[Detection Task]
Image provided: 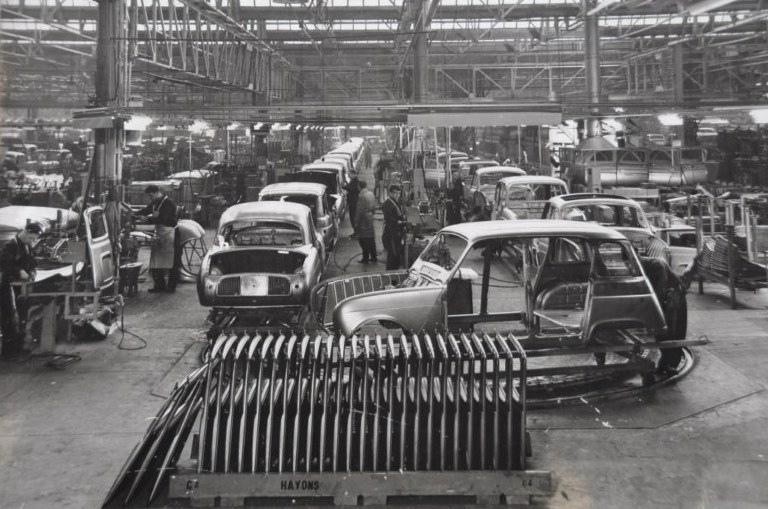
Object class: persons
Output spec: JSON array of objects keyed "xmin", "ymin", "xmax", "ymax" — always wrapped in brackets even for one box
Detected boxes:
[
  {"xmin": 344, "ymin": 143, "xmax": 405, "ymax": 271},
  {"xmin": 0, "ymin": 221, "xmax": 42, "ymax": 346},
  {"xmin": 133, "ymin": 184, "xmax": 177, "ymax": 294},
  {"xmin": 622, "ymin": 245, "xmax": 688, "ymax": 379}
]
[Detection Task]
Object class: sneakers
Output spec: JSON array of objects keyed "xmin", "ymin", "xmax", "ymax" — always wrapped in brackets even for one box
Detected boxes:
[
  {"xmin": 357, "ymin": 257, "xmax": 378, "ymax": 265},
  {"xmin": 148, "ymin": 284, "xmax": 176, "ymax": 294}
]
